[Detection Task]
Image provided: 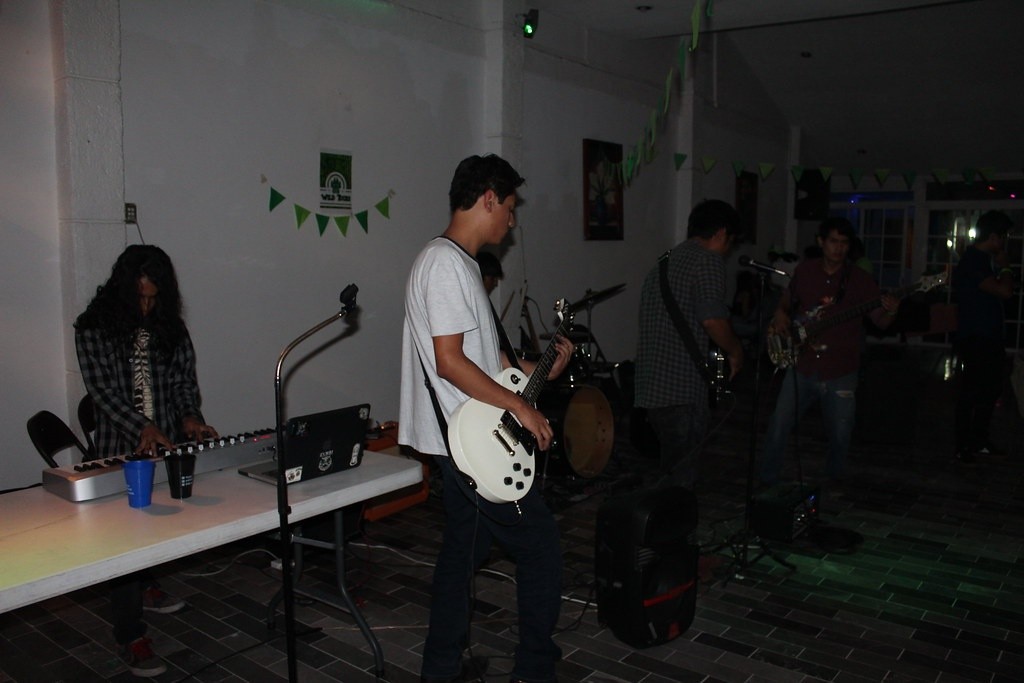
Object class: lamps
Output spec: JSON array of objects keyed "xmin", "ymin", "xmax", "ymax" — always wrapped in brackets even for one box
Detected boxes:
[{"xmin": 520, "ymin": 7, "xmax": 538, "ymax": 39}]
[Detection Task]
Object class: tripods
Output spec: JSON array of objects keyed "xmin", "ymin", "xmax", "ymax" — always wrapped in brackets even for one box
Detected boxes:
[{"xmin": 699, "ymin": 269, "xmax": 797, "ymax": 588}]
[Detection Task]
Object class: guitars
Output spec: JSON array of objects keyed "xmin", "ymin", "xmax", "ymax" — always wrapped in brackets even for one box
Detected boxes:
[
  {"xmin": 445, "ymin": 295, "xmax": 576, "ymax": 503},
  {"xmin": 706, "ymin": 343, "xmax": 735, "ymax": 414},
  {"xmin": 765, "ymin": 269, "xmax": 953, "ymax": 370}
]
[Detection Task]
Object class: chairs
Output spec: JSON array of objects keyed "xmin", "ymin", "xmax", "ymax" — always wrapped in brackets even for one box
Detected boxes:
[
  {"xmin": 78, "ymin": 394, "xmax": 96, "ymax": 454},
  {"xmin": 27, "ymin": 411, "xmax": 89, "ymax": 469}
]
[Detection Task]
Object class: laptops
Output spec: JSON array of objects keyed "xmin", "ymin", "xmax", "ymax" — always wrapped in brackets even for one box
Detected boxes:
[{"xmin": 238, "ymin": 403, "xmax": 372, "ymax": 486}]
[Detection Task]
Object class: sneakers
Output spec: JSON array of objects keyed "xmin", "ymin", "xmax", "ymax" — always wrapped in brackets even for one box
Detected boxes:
[
  {"xmin": 115, "ymin": 636, "xmax": 167, "ymax": 677},
  {"xmin": 421, "ymin": 655, "xmax": 490, "ymax": 683},
  {"xmin": 955, "ymin": 447, "xmax": 975, "ymax": 462},
  {"xmin": 142, "ymin": 586, "xmax": 185, "ymax": 613},
  {"xmin": 510, "ymin": 679, "xmax": 558, "ymax": 683},
  {"xmin": 971, "ymin": 442, "xmax": 1008, "ymax": 459}
]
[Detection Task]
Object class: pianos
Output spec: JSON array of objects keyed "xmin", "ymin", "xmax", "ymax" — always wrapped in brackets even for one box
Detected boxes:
[{"xmin": 42, "ymin": 422, "xmax": 296, "ymax": 500}]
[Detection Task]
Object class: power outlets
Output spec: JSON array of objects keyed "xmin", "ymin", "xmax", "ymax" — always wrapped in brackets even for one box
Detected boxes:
[{"xmin": 125, "ymin": 203, "xmax": 137, "ymax": 223}]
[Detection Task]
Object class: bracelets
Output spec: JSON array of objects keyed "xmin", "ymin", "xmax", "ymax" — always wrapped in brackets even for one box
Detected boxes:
[
  {"xmin": 1000, "ymin": 268, "xmax": 1013, "ymax": 274},
  {"xmin": 887, "ymin": 310, "xmax": 898, "ymax": 316}
]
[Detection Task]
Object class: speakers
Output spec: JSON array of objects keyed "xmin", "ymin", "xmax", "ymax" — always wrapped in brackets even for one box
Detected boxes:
[
  {"xmin": 596, "ymin": 484, "xmax": 699, "ymax": 648},
  {"xmin": 793, "ymin": 169, "xmax": 831, "ymax": 221}
]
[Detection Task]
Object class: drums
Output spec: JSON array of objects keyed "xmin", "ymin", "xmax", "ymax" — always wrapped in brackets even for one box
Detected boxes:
[
  {"xmin": 585, "ymin": 362, "xmax": 622, "ymax": 401},
  {"xmin": 536, "ymin": 383, "xmax": 616, "ymax": 479}
]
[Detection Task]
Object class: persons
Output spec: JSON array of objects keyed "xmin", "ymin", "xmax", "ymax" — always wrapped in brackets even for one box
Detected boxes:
[
  {"xmin": 950, "ymin": 209, "xmax": 1014, "ymax": 462},
  {"xmin": 74, "ymin": 245, "xmax": 220, "ymax": 677},
  {"xmin": 634, "ymin": 200, "xmax": 901, "ymax": 546},
  {"xmin": 397, "ymin": 154, "xmax": 576, "ymax": 683}
]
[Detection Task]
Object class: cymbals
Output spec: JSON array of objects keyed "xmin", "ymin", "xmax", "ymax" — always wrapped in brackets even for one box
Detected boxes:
[{"xmin": 566, "ymin": 284, "xmax": 628, "ymax": 314}]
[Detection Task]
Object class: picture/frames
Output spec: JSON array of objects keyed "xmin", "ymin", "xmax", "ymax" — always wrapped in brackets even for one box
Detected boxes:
[
  {"xmin": 736, "ymin": 170, "xmax": 759, "ymax": 244},
  {"xmin": 583, "ymin": 138, "xmax": 624, "ymax": 241}
]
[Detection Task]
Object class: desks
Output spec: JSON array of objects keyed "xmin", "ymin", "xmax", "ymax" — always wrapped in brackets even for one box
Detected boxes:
[
  {"xmin": 0, "ymin": 450, "xmax": 423, "ymax": 678},
  {"xmin": 365, "ymin": 420, "xmax": 430, "ymax": 521}
]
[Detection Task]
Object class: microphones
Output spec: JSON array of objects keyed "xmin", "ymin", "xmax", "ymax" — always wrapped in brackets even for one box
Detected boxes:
[{"xmin": 738, "ymin": 254, "xmax": 789, "ymax": 276}]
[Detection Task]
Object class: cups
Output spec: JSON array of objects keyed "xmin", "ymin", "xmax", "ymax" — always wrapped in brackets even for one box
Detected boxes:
[
  {"xmin": 163, "ymin": 454, "xmax": 196, "ymax": 499},
  {"xmin": 122, "ymin": 460, "xmax": 156, "ymax": 508}
]
